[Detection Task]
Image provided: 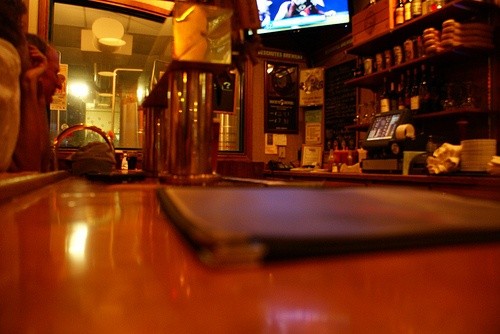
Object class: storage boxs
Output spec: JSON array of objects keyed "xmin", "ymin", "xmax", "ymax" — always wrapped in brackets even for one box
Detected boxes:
[{"xmin": 460, "ymin": 137, "xmax": 497, "ymax": 173}]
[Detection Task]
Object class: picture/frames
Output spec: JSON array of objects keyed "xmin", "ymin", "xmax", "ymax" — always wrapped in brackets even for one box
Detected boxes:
[
  {"xmin": 362, "ymin": 108, "xmax": 411, "ymax": 148},
  {"xmin": 263, "ymin": 59, "xmax": 300, "ymax": 135}
]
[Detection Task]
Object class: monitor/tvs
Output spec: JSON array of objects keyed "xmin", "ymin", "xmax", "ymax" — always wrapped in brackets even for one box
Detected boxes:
[
  {"xmin": 255, "ymin": 0, "xmax": 351, "ymax": 34},
  {"xmin": 364, "ymin": 109, "xmax": 405, "ymax": 143}
]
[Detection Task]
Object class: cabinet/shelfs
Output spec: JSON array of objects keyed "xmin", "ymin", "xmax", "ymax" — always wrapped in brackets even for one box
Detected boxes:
[{"xmin": 345, "ymin": 0, "xmax": 492, "ymax": 133}]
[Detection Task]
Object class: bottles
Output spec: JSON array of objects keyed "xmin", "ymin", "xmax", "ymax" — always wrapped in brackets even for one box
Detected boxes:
[
  {"xmin": 121, "ymin": 151, "xmax": 128, "ymax": 169},
  {"xmin": 328, "ymin": 148, "xmax": 338, "ymax": 172},
  {"xmin": 379, "ymin": 61, "xmax": 441, "ymax": 114},
  {"xmin": 396, "ymin": 0, "xmax": 445, "ymax": 26},
  {"xmin": 363, "ymin": 32, "xmax": 425, "ymax": 75},
  {"xmin": 441, "ymin": 82, "xmax": 480, "ymax": 110}
]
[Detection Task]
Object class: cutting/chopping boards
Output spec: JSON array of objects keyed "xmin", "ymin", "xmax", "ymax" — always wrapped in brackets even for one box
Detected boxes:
[{"xmin": 424, "ymin": 19, "xmax": 493, "ymax": 52}]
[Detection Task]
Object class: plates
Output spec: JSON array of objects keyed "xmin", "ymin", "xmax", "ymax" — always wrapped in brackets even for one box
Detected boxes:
[{"xmin": 459, "ymin": 139, "xmax": 497, "ymax": 172}]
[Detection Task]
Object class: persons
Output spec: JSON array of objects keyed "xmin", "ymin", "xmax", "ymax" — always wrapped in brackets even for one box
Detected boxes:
[
  {"xmin": 0, "ymin": 0, "xmax": 64, "ymax": 176},
  {"xmin": 256, "ymin": 0, "xmax": 336, "ymax": 28}
]
